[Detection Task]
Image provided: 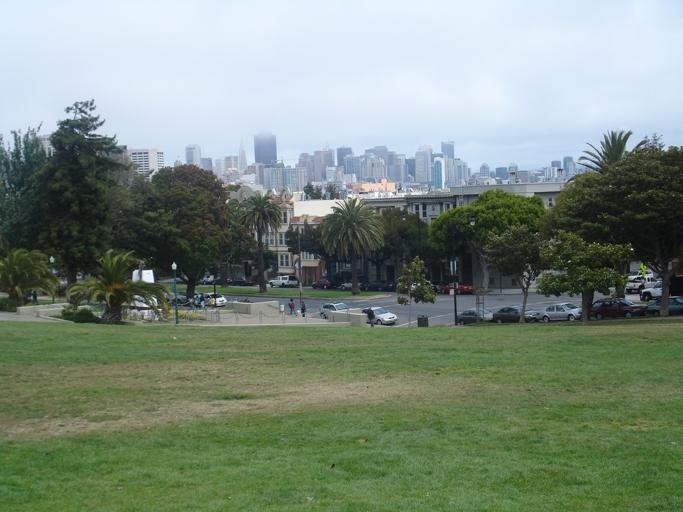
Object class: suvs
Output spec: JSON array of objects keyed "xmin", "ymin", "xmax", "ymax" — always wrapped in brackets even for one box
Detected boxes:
[{"xmin": 269, "ymin": 275, "xmax": 298, "ymax": 288}]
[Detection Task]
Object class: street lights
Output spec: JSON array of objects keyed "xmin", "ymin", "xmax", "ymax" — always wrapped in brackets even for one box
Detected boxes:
[
  {"xmin": 171, "ymin": 261, "xmax": 179, "ymax": 324},
  {"xmin": 49, "ymin": 256, "xmax": 55, "ymax": 303}
]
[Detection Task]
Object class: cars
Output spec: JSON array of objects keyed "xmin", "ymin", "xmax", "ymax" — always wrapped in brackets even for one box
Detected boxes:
[
  {"xmin": 176, "ymin": 278, "xmax": 253, "ymax": 286},
  {"xmin": 311, "ymin": 278, "xmax": 473, "ymax": 294},
  {"xmin": 165, "ymin": 291, "xmax": 227, "ymax": 307},
  {"xmin": 588, "ymin": 269, "xmax": 682, "ymax": 320},
  {"xmin": 362, "ymin": 306, "xmax": 397, "ymax": 325},
  {"xmin": 457, "ymin": 303, "xmax": 582, "ymax": 325},
  {"xmin": 320, "ymin": 303, "xmax": 352, "ymax": 320}
]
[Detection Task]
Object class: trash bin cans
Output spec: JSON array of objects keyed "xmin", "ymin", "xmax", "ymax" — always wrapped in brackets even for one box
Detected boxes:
[
  {"xmin": 280, "ymin": 305, "xmax": 284, "ymax": 312},
  {"xmin": 449, "ymin": 288, "xmax": 454, "ymax": 296},
  {"xmin": 418, "ymin": 314, "xmax": 428, "ymax": 327}
]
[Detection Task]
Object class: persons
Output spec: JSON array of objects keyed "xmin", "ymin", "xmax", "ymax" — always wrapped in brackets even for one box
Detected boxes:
[
  {"xmin": 366, "ymin": 307, "xmax": 375, "ymax": 326},
  {"xmin": 287, "ymin": 298, "xmax": 297, "ymax": 315},
  {"xmin": 300, "ymin": 301, "xmax": 306, "ymax": 317}
]
